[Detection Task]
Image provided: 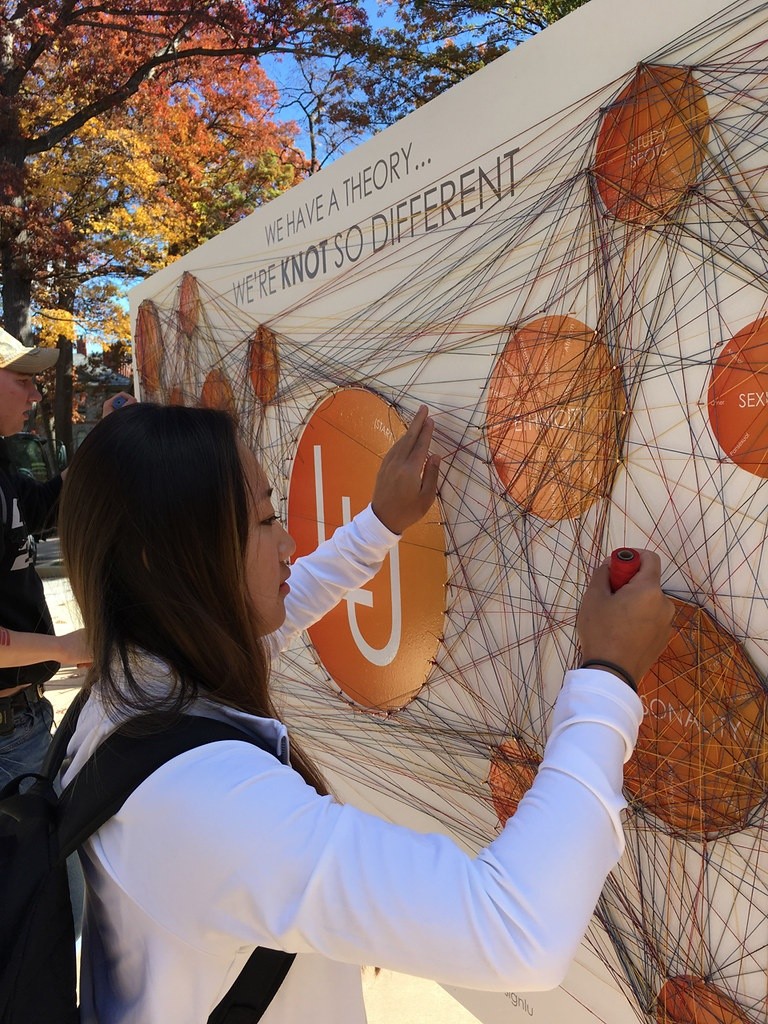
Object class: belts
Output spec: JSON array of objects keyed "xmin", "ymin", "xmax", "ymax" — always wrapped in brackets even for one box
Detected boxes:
[{"xmin": 0, "ymin": 680, "xmax": 47, "ymax": 713}]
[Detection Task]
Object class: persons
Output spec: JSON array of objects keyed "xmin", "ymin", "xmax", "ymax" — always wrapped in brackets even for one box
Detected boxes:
[
  {"xmin": 0, "ymin": 320, "xmax": 95, "ymax": 1024},
  {"xmin": 55, "ymin": 402, "xmax": 677, "ymax": 1024}
]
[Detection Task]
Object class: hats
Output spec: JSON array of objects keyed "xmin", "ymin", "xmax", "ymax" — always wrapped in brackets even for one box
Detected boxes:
[{"xmin": 0, "ymin": 326, "xmax": 60, "ymax": 373}]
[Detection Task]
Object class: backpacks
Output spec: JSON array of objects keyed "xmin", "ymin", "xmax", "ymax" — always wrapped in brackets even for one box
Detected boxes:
[{"xmin": 0, "ymin": 672, "xmax": 318, "ymax": 1024}]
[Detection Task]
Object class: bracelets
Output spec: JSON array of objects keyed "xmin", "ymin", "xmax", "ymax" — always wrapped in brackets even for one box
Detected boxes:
[{"xmin": 581, "ymin": 659, "xmax": 638, "ymax": 693}]
